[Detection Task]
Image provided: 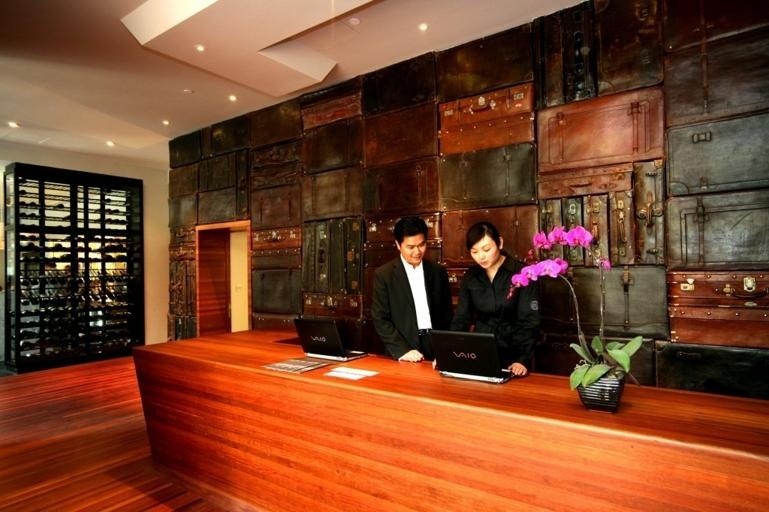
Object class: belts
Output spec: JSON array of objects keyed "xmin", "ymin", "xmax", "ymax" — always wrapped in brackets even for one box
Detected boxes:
[{"xmin": 417, "ymin": 328, "xmax": 434, "ymax": 338}]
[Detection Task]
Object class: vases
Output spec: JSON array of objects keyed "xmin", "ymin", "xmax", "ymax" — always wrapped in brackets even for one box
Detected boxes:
[{"xmin": 577, "ymin": 375, "xmax": 625, "ymax": 414}]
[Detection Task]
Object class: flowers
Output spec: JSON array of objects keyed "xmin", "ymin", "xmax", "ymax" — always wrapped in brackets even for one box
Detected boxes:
[{"xmin": 507, "ymin": 225, "xmax": 643, "ymax": 392}]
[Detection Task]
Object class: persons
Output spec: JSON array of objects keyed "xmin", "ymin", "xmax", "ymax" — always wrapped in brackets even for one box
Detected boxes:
[
  {"xmin": 448, "ymin": 220, "xmax": 543, "ymax": 377},
  {"xmin": 369, "ymin": 215, "xmax": 455, "ymax": 364}
]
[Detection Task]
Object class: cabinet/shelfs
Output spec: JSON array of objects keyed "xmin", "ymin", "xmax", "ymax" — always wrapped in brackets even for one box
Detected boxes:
[{"xmin": 5, "ymin": 162, "xmax": 144, "ymax": 375}]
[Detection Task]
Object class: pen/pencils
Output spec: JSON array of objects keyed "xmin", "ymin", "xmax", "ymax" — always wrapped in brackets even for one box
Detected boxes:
[{"xmin": 501, "ymin": 368, "xmax": 512, "ymax": 372}]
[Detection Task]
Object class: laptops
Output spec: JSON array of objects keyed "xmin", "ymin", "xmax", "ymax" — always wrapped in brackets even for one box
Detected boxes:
[
  {"xmin": 432, "ymin": 330, "xmax": 514, "ymax": 384},
  {"xmin": 293, "ymin": 319, "xmax": 368, "ymax": 361}
]
